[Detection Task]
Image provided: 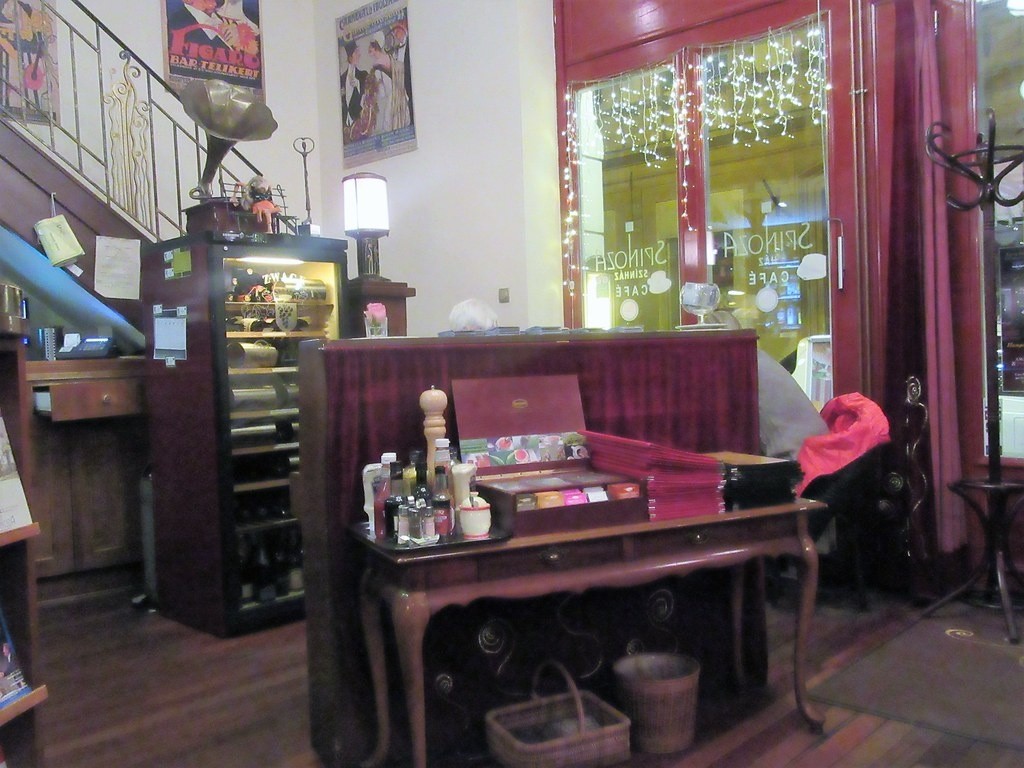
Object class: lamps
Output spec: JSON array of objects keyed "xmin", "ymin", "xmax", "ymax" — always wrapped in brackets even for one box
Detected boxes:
[{"xmin": 342, "ymin": 172, "xmax": 392, "ymax": 282}]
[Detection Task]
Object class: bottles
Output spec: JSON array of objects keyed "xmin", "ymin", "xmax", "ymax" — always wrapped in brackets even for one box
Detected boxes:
[
  {"xmin": 384, "ymin": 461, "xmax": 407, "ymax": 541},
  {"xmin": 431, "ymin": 466, "xmax": 455, "ymax": 536},
  {"xmin": 399, "ymin": 508, "xmax": 409, "ymax": 538},
  {"xmin": 372, "ymin": 453, "xmax": 396, "ymax": 540},
  {"xmin": 424, "ymin": 506, "xmax": 435, "ymax": 536},
  {"xmin": 412, "ymin": 460, "xmax": 432, "ymax": 499},
  {"xmin": 402, "ymin": 451, "xmax": 431, "ymax": 496},
  {"xmin": 431, "ymin": 494, "xmax": 451, "ymax": 538},
  {"xmin": 434, "ymin": 438, "xmax": 451, "ymax": 489},
  {"xmin": 236, "ymin": 530, "xmax": 304, "ymax": 602}
]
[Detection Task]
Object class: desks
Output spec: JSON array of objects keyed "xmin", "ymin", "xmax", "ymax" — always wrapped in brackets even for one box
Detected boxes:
[{"xmin": 345, "ymin": 496, "xmax": 828, "ymax": 768}]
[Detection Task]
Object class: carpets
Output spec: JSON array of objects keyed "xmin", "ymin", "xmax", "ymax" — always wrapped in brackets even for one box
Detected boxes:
[{"xmin": 805, "ymin": 600, "xmax": 1024, "ymax": 751}]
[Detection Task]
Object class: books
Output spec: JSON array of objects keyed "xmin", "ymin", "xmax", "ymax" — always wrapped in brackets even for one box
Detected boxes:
[
  {"xmin": 0, "ymin": 416, "xmax": 34, "ymax": 712},
  {"xmin": 35, "ymin": 214, "xmax": 86, "ymax": 267},
  {"xmin": 577, "ymin": 429, "xmax": 804, "ymax": 523}
]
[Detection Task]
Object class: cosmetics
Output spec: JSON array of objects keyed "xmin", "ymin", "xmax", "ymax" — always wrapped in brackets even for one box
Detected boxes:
[{"xmin": 363, "ymin": 382, "xmax": 492, "ymax": 547}]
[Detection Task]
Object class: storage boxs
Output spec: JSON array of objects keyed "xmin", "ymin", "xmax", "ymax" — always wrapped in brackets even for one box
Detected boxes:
[{"xmin": 451, "ymin": 374, "xmax": 648, "ymax": 536}]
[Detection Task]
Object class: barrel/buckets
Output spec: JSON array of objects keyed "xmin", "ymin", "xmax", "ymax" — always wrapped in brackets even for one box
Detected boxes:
[{"xmin": 614, "ymin": 652, "xmax": 701, "ymax": 754}]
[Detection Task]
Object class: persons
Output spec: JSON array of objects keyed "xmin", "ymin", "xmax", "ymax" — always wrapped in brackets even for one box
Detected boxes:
[{"xmin": 0, "ymin": 643, "xmax": 17, "ymax": 677}]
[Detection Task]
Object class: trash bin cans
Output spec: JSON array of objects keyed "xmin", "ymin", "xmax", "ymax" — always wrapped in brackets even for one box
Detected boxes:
[{"xmin": 611, "ymin": 651, "xmax": 702, "ymax": 755}]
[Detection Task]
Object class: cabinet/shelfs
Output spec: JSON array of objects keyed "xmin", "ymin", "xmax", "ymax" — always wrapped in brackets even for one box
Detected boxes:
[{"xmin": 0, "ymin": 336, "xmax": 48, "ymax": 768}]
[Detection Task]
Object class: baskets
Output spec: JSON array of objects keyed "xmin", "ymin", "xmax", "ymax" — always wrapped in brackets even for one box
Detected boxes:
[{"xmin": 486, "ymin": 654, "xmax": 632, "ymax": 768}]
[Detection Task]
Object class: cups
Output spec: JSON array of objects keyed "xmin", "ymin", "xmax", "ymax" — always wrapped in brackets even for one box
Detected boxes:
[
  {"xmin": 273, "ymin": 278, "xmax": 326, "ymax": 302},
  {"xmin": 231, "ymin": 386, "xmax": 277, "ymax": 411},
  {"xmin": 276, "ymin": 304, "xmax": 298, "ymax": 331},
  {"xmin": 229, "ymin": 340, "xmax": 278, "ymax": 368},
  {"xmin": 279, "ymin": 421, "xmax": 300, "ymax": 443},
  {"xmin": 364, "ymin": 316, "xmax": 388, "ymax": 339},
  {"xmin": 231, "ymin": 423, "xmax": 276, "ymax": 445},
  {"xmin": 452, "ymin": 462, "xmax": 478, "ymax": 506},
  {"xmin": 459, "ymin": 492, "xmax": 492, "ymax": 539},
  {"xmin": 281, "ymin": 383, "xmax": 299, "ymax": 407},
  {"xmin": 277, "ymin": 456, "xmax": 299, "ymax": 478}
]
